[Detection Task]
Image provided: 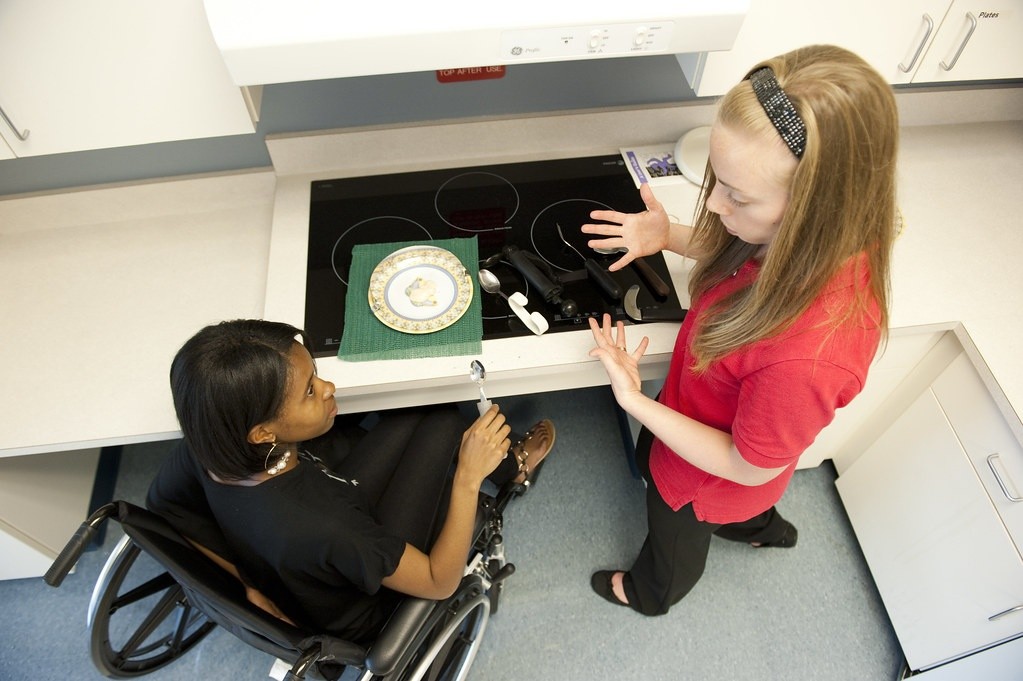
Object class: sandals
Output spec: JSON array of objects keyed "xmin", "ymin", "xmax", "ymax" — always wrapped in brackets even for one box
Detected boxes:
[{"xmin": 510, "ymin": 420, "xmax": 556, "ymax": 486}]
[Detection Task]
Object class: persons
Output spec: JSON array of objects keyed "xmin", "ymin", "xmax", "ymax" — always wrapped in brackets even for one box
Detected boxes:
[
  {"xmin": 581, "ymin": 44, "xmax": 899, "ymax": 618},
  {"xmin": 143, "ymin": 318, "xmax": 555, "ymax": 651}
]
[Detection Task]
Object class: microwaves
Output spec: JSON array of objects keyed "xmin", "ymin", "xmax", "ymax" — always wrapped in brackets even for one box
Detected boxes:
[{"xmin": 304, "ymin": 153, "xmax": 681, "ymax": 359}]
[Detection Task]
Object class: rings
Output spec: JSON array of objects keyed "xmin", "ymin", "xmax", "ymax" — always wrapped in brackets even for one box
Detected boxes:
[{"xmin": 617, "ymin": 347, "xmax": 626, "ymax": 350}]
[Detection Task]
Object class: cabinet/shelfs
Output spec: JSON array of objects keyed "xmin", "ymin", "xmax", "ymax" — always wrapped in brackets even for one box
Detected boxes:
[
  {"xmin": 835, "ymin": 349, "xmax": 1023, "ymax": 672},
  {"xmin": 794, "ymin": 322, "xmax": 947, "ymax": 470},
  {"xmin": 0, "ymin": 445, "xmax": 126, "ymax": 579},
  {"xmin": 674, "ymin": 0, "xmax": 1023, "ymax": 97},
  {"xmin": 901, "ymin": 636, "xmax": 1023, "ymax": 681},
  {"xmin": 0, "ymin": 0, "xmax": 264, "ymax": 161}
]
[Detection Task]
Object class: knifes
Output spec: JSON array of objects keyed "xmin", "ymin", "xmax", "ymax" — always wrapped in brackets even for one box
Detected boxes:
[{"xmin": 555, "ymin": 221, "xmax": 624, "ymax": 299}]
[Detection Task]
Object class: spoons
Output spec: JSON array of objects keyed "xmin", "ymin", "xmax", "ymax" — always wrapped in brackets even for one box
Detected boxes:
[
  {"xmin": 480, "ymin": 269, "xmax": 549, "ymax": 335},
  {"xmin": 471, "ymin": 360, "xmax": 509, "ymax": 460}
]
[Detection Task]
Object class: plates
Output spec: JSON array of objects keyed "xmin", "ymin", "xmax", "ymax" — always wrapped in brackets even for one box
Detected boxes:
[{"xmin": 368, "ymin": 245, "xmax": 474, "ymax": 335}]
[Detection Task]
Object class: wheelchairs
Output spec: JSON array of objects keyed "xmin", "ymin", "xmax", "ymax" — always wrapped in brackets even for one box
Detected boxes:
[{"xmin": 40, "ymin": 430, "xmax": 549, "ymax": 681}]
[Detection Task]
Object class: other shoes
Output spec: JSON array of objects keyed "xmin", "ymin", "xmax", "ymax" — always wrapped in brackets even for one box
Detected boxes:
[
  {"xmin": 713, "ymin": 521, "xmax": 798, "ymax": 547},
  {"xmin": 592, "ymin": 570, "xmax": 629, "ymax": 606}
]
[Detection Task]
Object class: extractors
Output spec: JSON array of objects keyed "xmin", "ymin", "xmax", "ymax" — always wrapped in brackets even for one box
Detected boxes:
[{"xmin": 204, "ymin": 0, "xmax": 746, "ymax": 88}]
